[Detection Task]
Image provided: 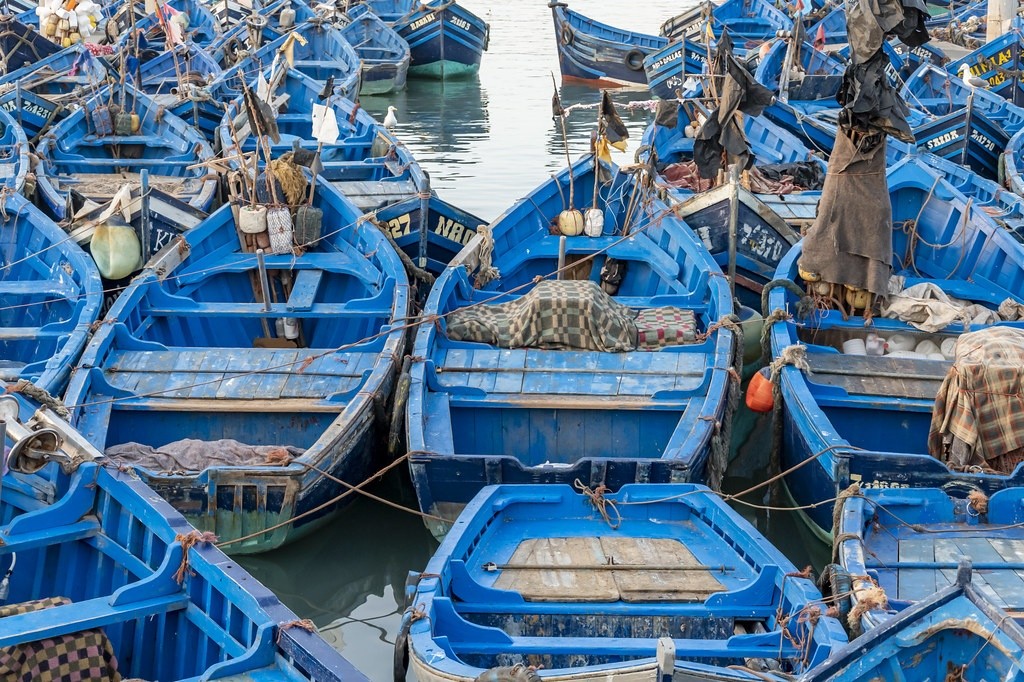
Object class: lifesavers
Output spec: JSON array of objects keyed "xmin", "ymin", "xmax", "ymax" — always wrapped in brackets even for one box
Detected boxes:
[
  {"xmin": 227, "ymin": 36, "xmax": 244, "ymax": 62},
  {"xmin": 560, "ymin": 27, "xmax": 572, "ymax": 44},
  {"xmin": 624, "ymin": 50, "xmax": 646, "ymax": 70}
]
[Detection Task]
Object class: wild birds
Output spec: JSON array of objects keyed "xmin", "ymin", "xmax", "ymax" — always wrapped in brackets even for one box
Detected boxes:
[{"xmin": 382, "ymin": 106, "xmax": 398, "ymax": 135}]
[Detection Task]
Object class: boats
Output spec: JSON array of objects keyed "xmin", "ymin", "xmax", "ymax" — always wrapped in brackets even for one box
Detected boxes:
[
  {"xmin": 794, "ymin": 558, "xmax": 1024, "ymax": 682},
  {"xmin": 58, "ymin": 168, "xmax": 408, "ymax": 561},
  {"xmin": 392, "ymin": 479, "xmax": 844, "ymax": 682},
  {"xmin": 0, "ymin": 0, "xmax": 1023, "ymax": 471},
  {"xmin": 0, "ymin": 376, "xmax": 370, "ymax": 682},
  {"xmin": 0, "ymin": 172, "xmax": 105, "ymax": 411},
  {"xmin": 763, "ymin": 135, "xmax": 1023, "ymax": 549},
  {"xmin": 833, "ymin": 484, "xmax": 1024, "ymax": 646},
  {"xmin": 404, "ymin": 154, "xmax": 734, "ymax": 546}
]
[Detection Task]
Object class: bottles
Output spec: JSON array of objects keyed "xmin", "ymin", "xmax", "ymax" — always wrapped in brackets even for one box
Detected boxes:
[
  {"xmin": 35, "ymin": 0, "xmax": 104, "ymax": 47},
  {"xmin": 843, "ymin": 332, "xmax": 958, "ymax": 361}
]
